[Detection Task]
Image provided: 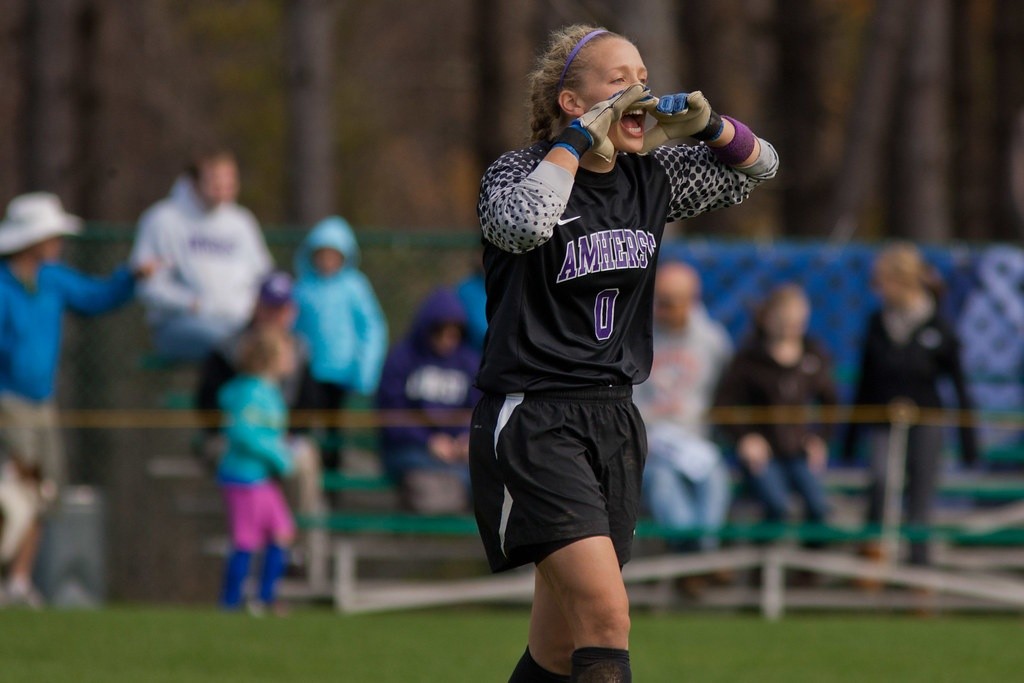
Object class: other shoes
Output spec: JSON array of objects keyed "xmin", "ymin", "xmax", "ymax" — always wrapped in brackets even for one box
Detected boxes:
[
  {"xmin": 677, "ymin": 576, "xmax": 728, "ymax": 609},
  {"xmin": 899, "ymin": 587, "xmax": 934, "ymax": 618},
  {"xmin": 847, "ymin": 543, "xmax": 882, "ymax": 594},
  {"xmin": 6, "ymin": 585, "xmax": 45, "ymax": 608}
]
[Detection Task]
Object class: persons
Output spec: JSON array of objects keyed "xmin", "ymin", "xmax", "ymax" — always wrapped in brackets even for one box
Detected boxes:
[
  {"xmin": 469, "ymin": 23, "xmax": 779, "ymax": 682},
  {"xmin": 630, "ymin": 236, "xmax": 981, "ymax": 616},
  {"xmin": 128, "ymin": 149, "xmax": 486, "ymax": 619},
  {"xmin": 0, "ymin": 184, "xmax": 155, "ymax": 615}
]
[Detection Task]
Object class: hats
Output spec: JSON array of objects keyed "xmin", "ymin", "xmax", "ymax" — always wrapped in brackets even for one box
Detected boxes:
[{"xmin": 0, "ymin": 193, "xmax": 82, "ymax": 256}]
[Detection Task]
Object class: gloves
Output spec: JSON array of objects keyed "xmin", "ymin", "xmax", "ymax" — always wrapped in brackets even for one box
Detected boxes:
[
  {"xmin": 552, "ymin": 82, "xmax": 659, "ymax": 163},
  {"xmin": 838, "ymin": 430, "xmax": 859, "ymax": 466},
  {"xmin": 636, "ymin": 90, "xmax": 724, "ymax": 156},
  {"xmin": 959, "ymin": 427, "xmax": 979, "ymax": 473}
]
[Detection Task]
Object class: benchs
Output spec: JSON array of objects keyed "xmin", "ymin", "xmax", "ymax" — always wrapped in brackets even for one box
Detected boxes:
[{"xmin": 128, "ymin": 342, "xmax": 1024, "ymax": 614}]
